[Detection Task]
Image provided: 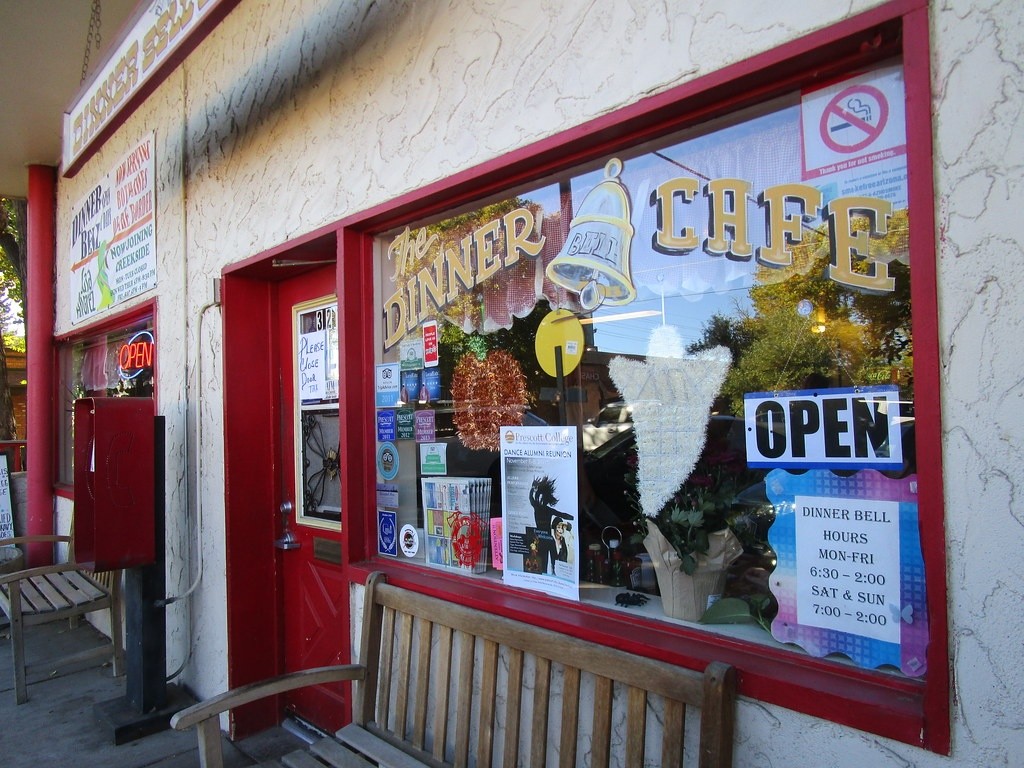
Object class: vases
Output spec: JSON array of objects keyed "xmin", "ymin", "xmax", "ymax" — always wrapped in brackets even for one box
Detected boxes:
[{"xmin": 640, "ymin": 521, "xmax": 742, "ymax": 622}]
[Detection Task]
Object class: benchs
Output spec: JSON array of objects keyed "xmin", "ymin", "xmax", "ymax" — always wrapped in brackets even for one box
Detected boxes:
[
  {"xmin": 0, "ymin": 509, "xmax": 125, "ymax": 708},
  {"xmin": 170, "ymin": 571, "xmax": 740, "ymax": 768}
]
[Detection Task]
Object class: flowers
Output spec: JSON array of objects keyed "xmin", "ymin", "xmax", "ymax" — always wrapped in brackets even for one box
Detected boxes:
[{"xmin": 616, "ymin": 430, "xmax": 757, "ymax": 573}]
